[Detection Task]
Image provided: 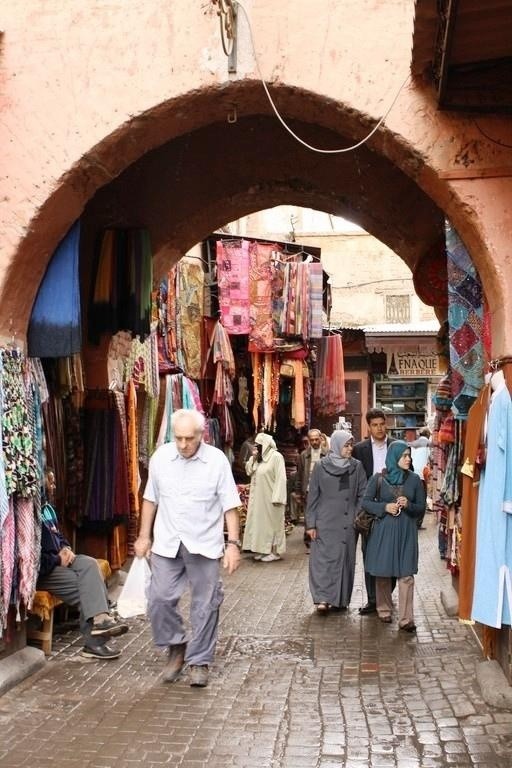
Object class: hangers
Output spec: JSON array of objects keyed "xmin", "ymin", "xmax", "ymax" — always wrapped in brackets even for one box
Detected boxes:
[
  {"xmin": 108, "ymin": 367, "xmax": 126, "ymax": 393},
  {"xmin": 318, "ymin": 322, "xmax": 337, "ymax": 338},
  {"xmin": 327, "ymin": 324, "xmax": 343, "ymax": 334},
  {"xmin": 215, "ymin": 227, "xmax": 323, "ymax": 269},
  {"xmin": 5, "ymin": 325, "xmax": 22, "ymax": 352},
  {"xmin": 477, "ymin": 360, "xmax": 508, "ymax": 406}
]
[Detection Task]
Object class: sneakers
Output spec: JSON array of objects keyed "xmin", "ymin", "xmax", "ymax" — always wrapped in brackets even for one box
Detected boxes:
[
  {"xmin": 254, "ymin": 554, "xmax": 264, "ymax": 560},
  {"xmin": 90, "ymin": 616, "xmax": 129, "ymax": 637},
  {"xmin": 261, "ymin": 553, "xmax": 281, "ymax": 562},
  {"xmin": 81, "ymin": 643, "xmax": 122, "ymax": 660},
  {"xmin": 162, "ymin": 646, "xmax": 187, "ymax": 682},
  {"xmin": 316, "ymin": 602, "xmax": 329, "ymax": 615},
  {"xmin": 189, "ymin": 664, "xmax": 210, "ymax": 687}
]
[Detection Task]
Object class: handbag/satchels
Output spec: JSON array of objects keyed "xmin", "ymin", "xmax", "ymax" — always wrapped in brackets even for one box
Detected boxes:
[{"xmin": 350, "ymin": 476, "xmax": 382, "ymax": 533}]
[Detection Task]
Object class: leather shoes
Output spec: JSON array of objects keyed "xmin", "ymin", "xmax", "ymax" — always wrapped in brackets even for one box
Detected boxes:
[
  {"xmin": 359, "ymin": 603, "xmax": 376, "ymax": 614},
  {"xmin": 398, "ymin": 621, "xmax": 416, "ymax": 631},
  {"xmin": 381, "ymin": 616, "xmax": 392, "ymax": 623}
]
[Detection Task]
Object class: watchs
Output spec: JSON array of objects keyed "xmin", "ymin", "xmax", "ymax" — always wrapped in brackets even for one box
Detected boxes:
[{"xmin": 226, "ymin": 540, "xmax": 242, "ymax": 548}]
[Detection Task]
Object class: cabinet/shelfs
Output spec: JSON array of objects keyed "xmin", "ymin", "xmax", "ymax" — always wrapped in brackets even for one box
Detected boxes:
[{"xmin": 375, "ymin": 395, "xmax": 428, "ymax": 431}]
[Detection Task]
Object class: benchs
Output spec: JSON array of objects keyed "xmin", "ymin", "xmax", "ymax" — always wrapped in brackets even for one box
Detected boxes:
[{"xmin": 16, "ymin": 557, "xmax": 114, "ymax": 654}]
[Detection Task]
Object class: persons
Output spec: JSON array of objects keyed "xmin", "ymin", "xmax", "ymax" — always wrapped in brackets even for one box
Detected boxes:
[
  {"xmin": 38, "ymin": 464, "xmax": 129, "ymax": 659},
  {"xmin": 294, "ymin": 429, "xmax": 328, "ymax": 554},
  {"xmin": 132, "ymin": 409, "xmax": 243, "ymax": 688},
  {"xmin": 410, "ymin": 427, "xmax": 432, "ymax": 530},
  {"xmin": 241, "ymin": 432, "xmax": 286, "ymax": 562},
  {"xmin": 305, "ymin": 429, "xmax": 368, "ymax": 614},
  {"xmin": 361, "ymin": 440, "xmax": 426, "ymax": 632},
  {"xmin": 353, "ymin": 407, "xmax": 397, "ymax": 613}
]
